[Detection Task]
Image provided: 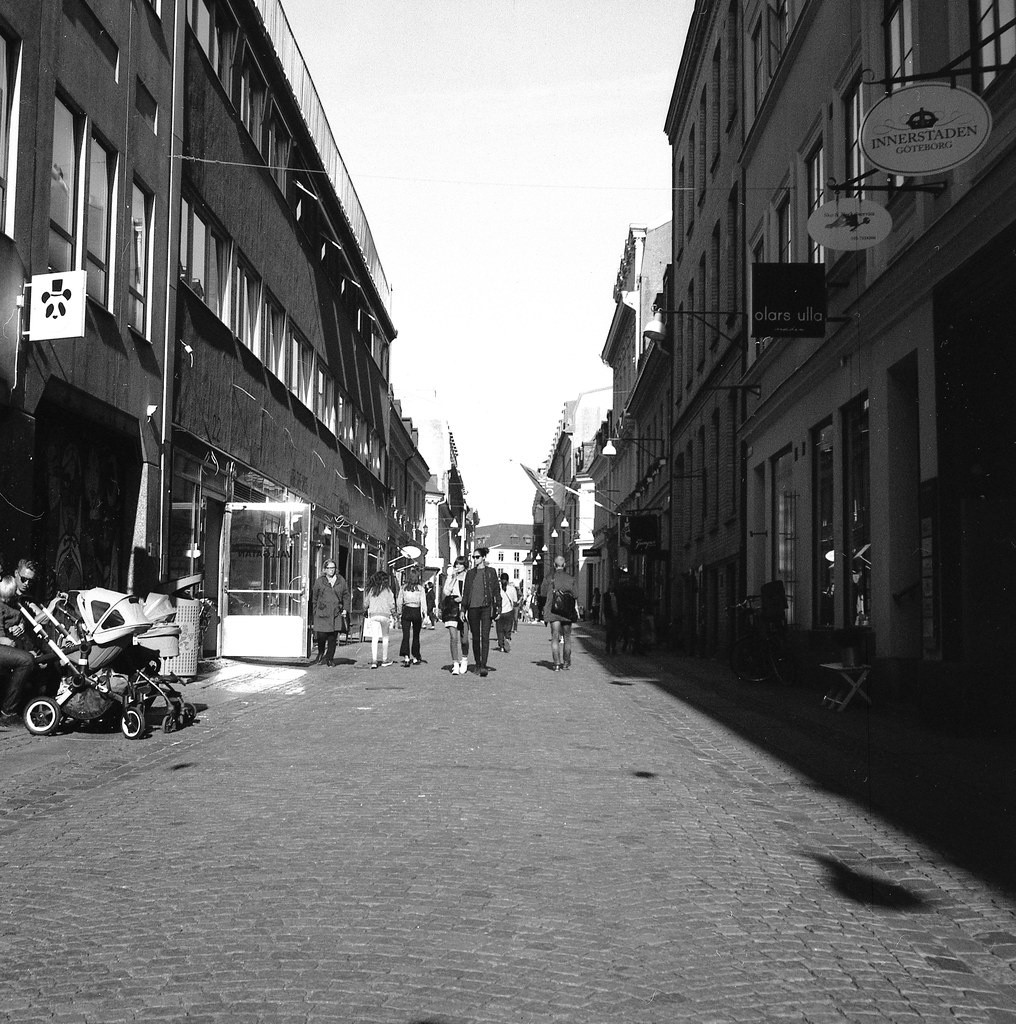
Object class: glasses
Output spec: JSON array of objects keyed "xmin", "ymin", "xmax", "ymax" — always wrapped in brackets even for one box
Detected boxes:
[
  {"xmin": 18, "ymin": 571, "xmax": 34, "ymax": 584},
  {"xmin": 472, "ymin": 555, "xmax": 483, "ymax": 559},
  {"xmin": 325, "ymin": 566, "xmax": 337, "ymax": 570}
]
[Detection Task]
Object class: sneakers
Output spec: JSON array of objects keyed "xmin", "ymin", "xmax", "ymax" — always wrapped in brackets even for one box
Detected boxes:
[{"xmin": 0, "ymin": 712, "xmax": 28, "ymax": 732}]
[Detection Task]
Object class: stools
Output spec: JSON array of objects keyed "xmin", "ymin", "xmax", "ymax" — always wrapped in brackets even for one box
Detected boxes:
[{"xmin": 820, "ymin": 661, "xmax": 874, "ymax": 712}]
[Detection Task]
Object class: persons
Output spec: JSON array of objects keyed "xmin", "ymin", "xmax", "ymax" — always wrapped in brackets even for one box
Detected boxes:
[
  {"xmin": 602, "ymin": 580, "xmax": 653, "ymax": 656},
  {"xmin": 397, "ymin": 574, "xmax": 427, "ymax": 667},
  {"xmin": 459, "ymin": 548, "xmax": 502, "ymax": 677},
  {"xmin": 591, "ymin": 587, "xmax": 601, "ymax": 625},
  {"xmin": 443, "ymin": 556, "xmax": 470, "ymax": 675},
  {"xmin": 313, "ymin": 559, "xmax": 350, "ymax": 668},
  {"xmin": 362, "ymin": 571, "xmax": 395, "ymax": 669},
  {"xmin": 495, "ymin": 573, "xmax": 523, "ymax": 654},
  {"xmin": 0, "ymin": 558, "xmax": 61, "ymax": 731},
  {"xmin": 520, "ymin": 587, "xmax": 539, "ymax": 625},
  {"xmin": 426, "ymin": 582, "xmax": 443, "ymax": 630},
  {"xmin": 541, "ymin": 556, "xmax": 579, "ymax": 672}
]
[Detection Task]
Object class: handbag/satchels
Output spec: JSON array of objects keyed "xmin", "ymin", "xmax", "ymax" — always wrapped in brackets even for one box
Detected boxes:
[
  {"xmin": 551, "ymin": 589, "xmax": 577, "ymax": 622},
  {"xmin": 340, "ymin": 616, "xmax": 350, "ymax": 635},
  {"xmin": 363, "ymin": 618, "xmax": 383, "ymax": 640}
]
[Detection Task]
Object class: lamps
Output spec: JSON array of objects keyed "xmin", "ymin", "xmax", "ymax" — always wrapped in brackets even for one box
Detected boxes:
[
  {"xmin": 577, "ymin": 489, "xmax": 620, "ymax": 502},
  {"xmin": 439, "ymin": 517, "xmax": 458, "ymax": 527},
  {"xmin": 644, "ymin": 305, "xmax": 749, "ymax": 342},
  {"xmin": 602, "ymin": 438, "xmax": 665, "ymax": 455},
  {"xmin": 560, "ymin": 516, "xmax": 595, "ymax": 527},
  {"xmin": 532, "ymin": 558, "xmax": 553, "ymax": 566},
  {"xmin": 550, "ymin": 528, "xmax": 578, "ymax": 537},
  {"xmin": 535, "ymin": 553, "xmax": 558, "ymax": 560},
  {"xmin": 542, "ymin": 543, "xmax": 560, "ymax": 551}
]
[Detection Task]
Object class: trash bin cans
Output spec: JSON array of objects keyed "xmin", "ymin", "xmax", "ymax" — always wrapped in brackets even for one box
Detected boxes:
[{"xmin": 150, "ymin": 571, "xmax": 204, "ymax": 678}]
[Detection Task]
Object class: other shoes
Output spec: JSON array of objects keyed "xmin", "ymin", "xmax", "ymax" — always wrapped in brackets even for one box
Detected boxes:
[
  {"xmin": 370, "ymin": 661, "xmax": 394, "ymax": 669},
  {"xmin": 553, "ymin": 662, "xmax": 570, "ymax": 671},
  {"xmin": 499, "ymin": 639, "xmax": 510, "ymax": 653},
  {"xmin": 316, "ymin": 651, "xmax": 336, "ymax": 667},
  {"xmin": 473, "ymin": 662, "xmax": 488, "ymax": 677},
  {"xmin": 623, "ymin": 646, "xmax": 633, "ymax": 653},
  {"xmin": 403, "ymin": 658, "xmax": 421, "ymax": 667},
  {"xmin": 605, "ymin": 647, "xmax": 616, "ymax": 655},
  {"xmin": 452, "ymin": 659, "xmax": 469, "ymax": 675}
]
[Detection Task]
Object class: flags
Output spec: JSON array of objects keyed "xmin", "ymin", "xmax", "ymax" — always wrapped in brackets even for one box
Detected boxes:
[{"xmin": 521, "ymin": 464, "xmax": 566, "ymax": 511}]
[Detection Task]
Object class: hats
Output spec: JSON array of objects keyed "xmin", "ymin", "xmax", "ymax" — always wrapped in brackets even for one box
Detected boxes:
[{"xmin": 555, "ymin": 556, "xmax": 565, "ymax": 566}]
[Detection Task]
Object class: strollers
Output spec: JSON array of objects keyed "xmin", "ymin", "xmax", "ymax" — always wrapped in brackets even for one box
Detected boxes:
[
  {"xmin": 15, "ymin": 587, "xmax": 195, "ymax": 737},
  {"xmin": 518, "ymin": 603, "xmax": 531, "ymax": 623},
  {"xmin": 623, "ymin": 616, "xmax": 655, "ymax": 654}
]
